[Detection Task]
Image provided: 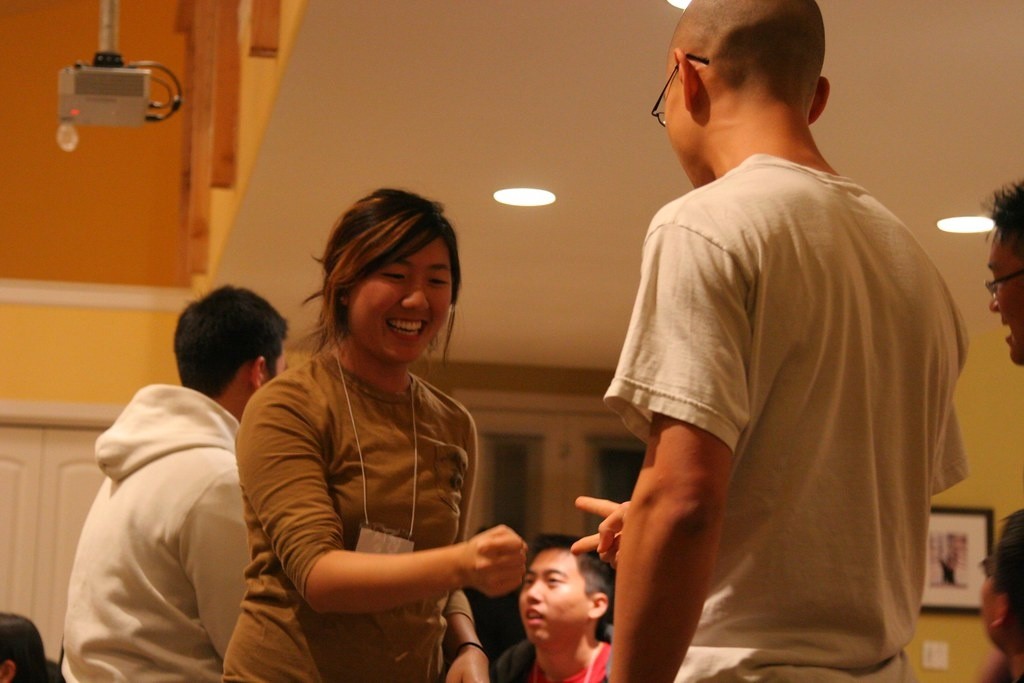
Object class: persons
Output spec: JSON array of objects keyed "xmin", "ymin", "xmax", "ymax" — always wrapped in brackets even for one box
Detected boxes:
[
  {"xmin": 979, "ymin": 508, "xmax": 1024, "ymax": 683},
  {"xmin": 570, "ymin": 0, "xmax": 972, "ymax": 683},
  {"xmin": 222, "ymin": 186, "xmax": 529, "ymax": 683},
  {"xmin": 490, "ymin": 532, "xmax": 612, "ymax": 683},
  {"xmin": 980, "ymin": 178, "xmax": 1024, "ymax": 366},
  {"xmin": 0, "ymin": 613, "xmax": 49, "ymax": 683},
  {"xmin": 60, "ymin": 283, "xmax": 289, "ymax": 683}
]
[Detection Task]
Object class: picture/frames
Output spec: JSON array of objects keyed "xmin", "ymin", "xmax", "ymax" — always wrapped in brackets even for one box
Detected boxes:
[{"xmin": 922, "ymin": 506, "xmax": 995, "ymax": 614}]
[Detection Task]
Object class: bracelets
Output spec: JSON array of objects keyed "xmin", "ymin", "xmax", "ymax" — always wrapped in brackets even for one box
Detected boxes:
[{"xmin": 455, "ymin": 642, "xmax": 489, "ymax": 658}]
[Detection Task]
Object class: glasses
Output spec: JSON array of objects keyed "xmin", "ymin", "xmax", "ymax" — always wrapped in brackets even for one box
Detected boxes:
[
  {"xmin": 651, "ymin": 53, "xmax": 709, "ymax": 128},
  {"xmin": 986, "ymin": 269, "xmax": 1024, "ymax": 296}
]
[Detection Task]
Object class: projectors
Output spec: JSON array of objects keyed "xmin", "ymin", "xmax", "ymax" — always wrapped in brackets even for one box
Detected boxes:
[{"xmin": 58, "ymin": 62, "xmax": 152, "ymax": 126}]
